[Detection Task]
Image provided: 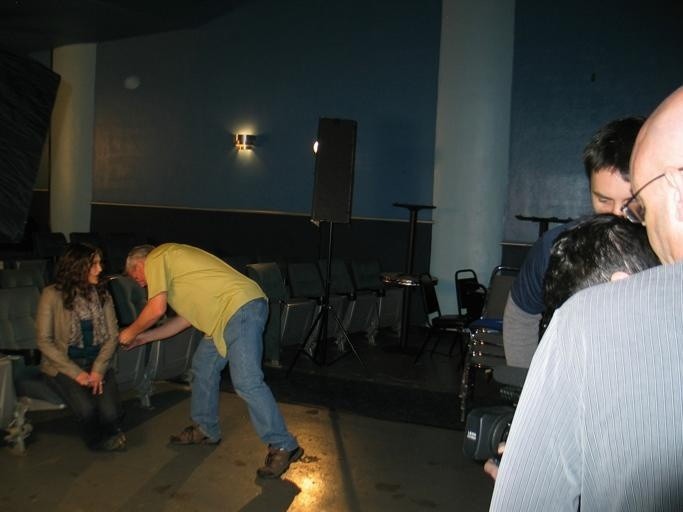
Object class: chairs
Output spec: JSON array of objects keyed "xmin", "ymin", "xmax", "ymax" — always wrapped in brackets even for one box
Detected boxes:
[
  {"xmin": 417, "ymin": 271, "xmax": 458, "ymax": 368},
  {"xmin": 482, "ymin": 265, "xmax": 517, "ymax": 319},
  {"xmin": 454, "ymin": 267, "xmax": 484, "ymax": 366},
  {"xmin": 0, "ymin": 254, "xmax": 402, "ymax": 430}
]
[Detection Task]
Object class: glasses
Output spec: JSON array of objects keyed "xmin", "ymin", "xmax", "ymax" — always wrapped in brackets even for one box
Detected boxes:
[{"xmin": 620, "ymin": 165, "xmax": 681, "ymax": 223}]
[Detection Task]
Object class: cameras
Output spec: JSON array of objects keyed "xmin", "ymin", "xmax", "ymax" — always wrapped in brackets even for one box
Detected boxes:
[{"xmin": 464, "ymin": 402, "xmax": 516, "ymax": 462}]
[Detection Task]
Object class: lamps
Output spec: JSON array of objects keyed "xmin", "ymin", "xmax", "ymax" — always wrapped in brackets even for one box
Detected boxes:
[{"xmin": 234, "ymin": 130, "xmax": 260, "ymax": 152}]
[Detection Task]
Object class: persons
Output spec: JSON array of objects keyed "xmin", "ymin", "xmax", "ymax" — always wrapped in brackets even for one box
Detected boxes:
[
  {"xmin": 119, "ymin": 243, "xmax": 304, "ymax": 481},
  {"xmin": 35, "ymin": 241, "xmax": 126, "ymax": 452},
  {"xmin": 483, "ymin": 214, "xmax": 661, "ymax": 482},
  {"xmin": 502, "ymin": 113, "xmax": 648, "ymax": 372},
  {"xmin": 488, "ymin": 84, "xmax": 683, "ymax": 511}
]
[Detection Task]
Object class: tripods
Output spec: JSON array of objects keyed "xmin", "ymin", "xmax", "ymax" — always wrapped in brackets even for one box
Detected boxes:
[{"xmin": 286, "ymin": 231, "xmax": 368, "ymax": 377}]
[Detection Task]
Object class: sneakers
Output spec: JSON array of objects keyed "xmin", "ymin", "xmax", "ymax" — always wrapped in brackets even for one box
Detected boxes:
[
  {"xmin": 256, "ymin": 443, "xmax": 304, "ymax": 479},
  {"xmin": 169, "ymin": 425, "xmax": 220, "ymax": 445},
  {"xmin": 93, "ymin": 428, "xmax": 127, "ymax": 451}
]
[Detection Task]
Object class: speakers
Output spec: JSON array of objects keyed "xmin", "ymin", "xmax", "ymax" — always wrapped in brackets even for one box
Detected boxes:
[
  {"xmin": 310, "ymin": 117, "xmax": 358, "ymax": 224},
  {"xmin": 0, "ymin": 55, "xmax": 62, "ymax": 246}
]
